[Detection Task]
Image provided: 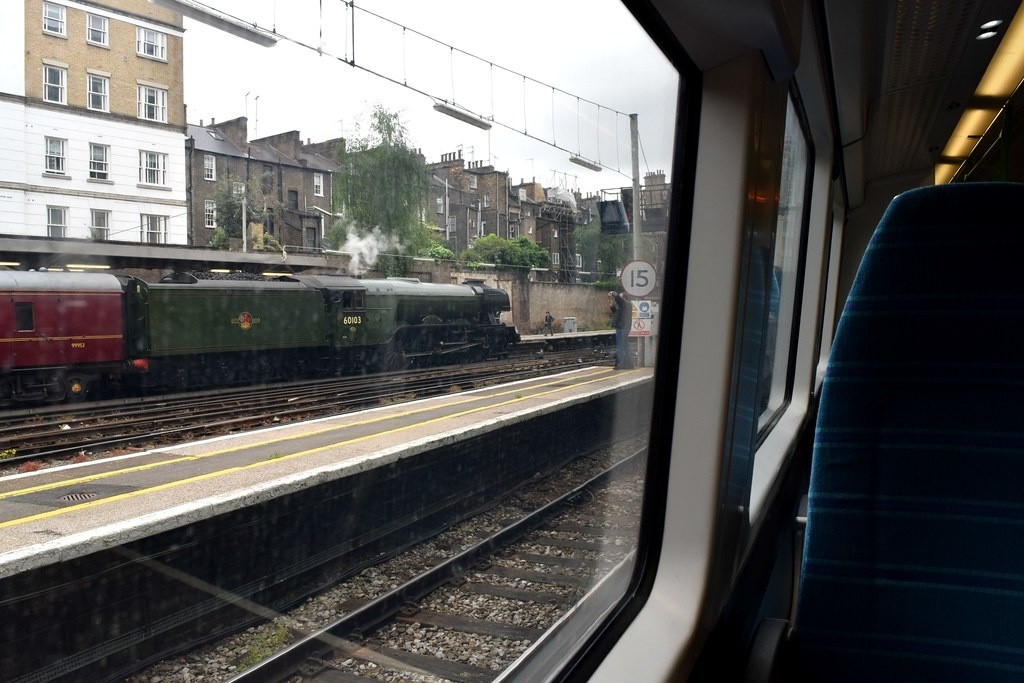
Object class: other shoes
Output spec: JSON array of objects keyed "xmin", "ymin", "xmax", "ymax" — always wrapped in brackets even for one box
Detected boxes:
[
  {"xmin": 623, "ymin": 366, "xmax": 632, "ymax": 369},
  {"xmin": 613, "ymin": 367, "xmax": 622, "ymax": 370}
]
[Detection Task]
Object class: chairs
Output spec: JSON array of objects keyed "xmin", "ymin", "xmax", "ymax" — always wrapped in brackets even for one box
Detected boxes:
[{"xmin": 776, "ymin": 182, "xmax": 1024, "ymax": 683}]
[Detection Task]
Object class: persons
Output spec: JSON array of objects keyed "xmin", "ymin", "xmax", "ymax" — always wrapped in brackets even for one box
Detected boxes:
[
  {"xmin": 544, "ymin": 312, "xmax": 555, "ymax": 336},
  {"xmin": 608, "ymin": 291, "xmax": 633, "ymax": 370}
]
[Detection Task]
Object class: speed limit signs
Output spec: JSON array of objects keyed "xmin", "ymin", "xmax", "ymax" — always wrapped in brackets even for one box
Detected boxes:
[{"xmin": 619, "ymin": 259, "xmax": 658, "ymax": 296}]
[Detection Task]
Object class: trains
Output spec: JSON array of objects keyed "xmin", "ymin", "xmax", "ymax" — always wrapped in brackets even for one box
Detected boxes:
[{"xmin": 0, "ymin": 266, "xmax": 521, "ymax": 408}]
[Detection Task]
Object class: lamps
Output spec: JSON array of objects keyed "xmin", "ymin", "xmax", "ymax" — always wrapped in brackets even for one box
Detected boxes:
[
  {"xmin": 433, "ymin": 103, "xmax": 506, "ymax": 133},
  {"xmin": 150, "ymin": 0, "xmax": 281, "ymax": 48},
  {"xmin": 569, "ymin": 156, "xmax": 602, "ymax": 172}
]
